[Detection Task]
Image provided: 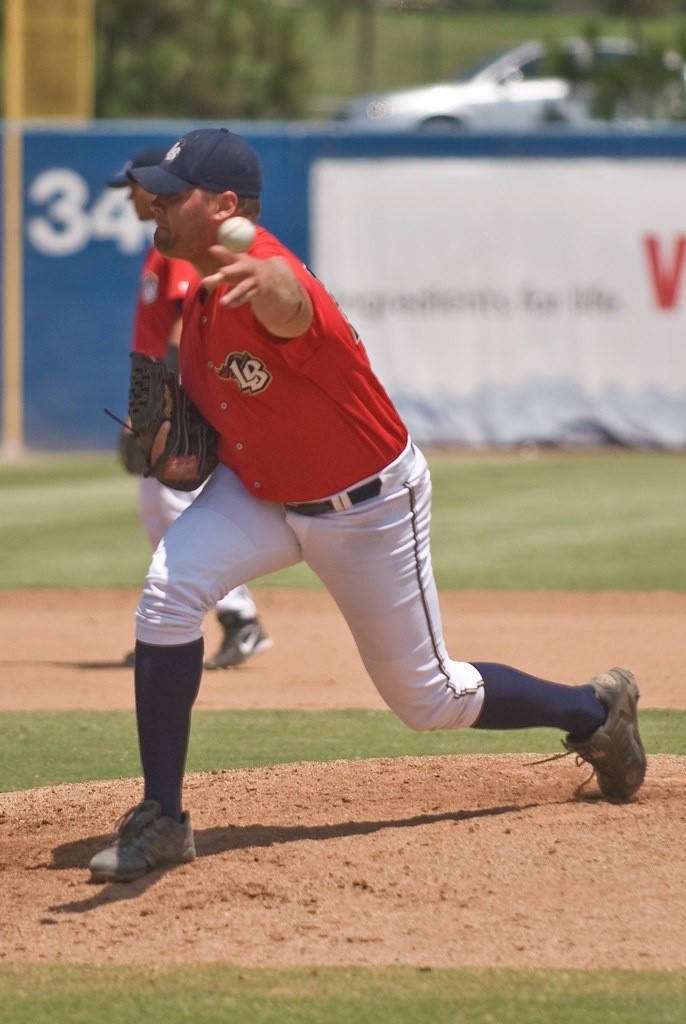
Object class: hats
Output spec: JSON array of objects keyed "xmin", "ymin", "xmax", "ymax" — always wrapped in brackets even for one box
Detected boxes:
[
  {"xmin": 127, "ymin": 128, "xmax": 262, "ymax": 200},
  {"xmin": 106, "ymin": 151, "xmax": 168, "ymax": 187}
]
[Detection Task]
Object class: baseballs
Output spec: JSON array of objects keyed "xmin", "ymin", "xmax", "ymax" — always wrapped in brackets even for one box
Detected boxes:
[{"xmin": 218, "ymin": 217, "xmax": 257, "ymax": 253}]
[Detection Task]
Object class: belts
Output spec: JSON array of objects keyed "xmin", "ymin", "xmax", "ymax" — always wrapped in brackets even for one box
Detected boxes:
[{"xmin": 285, "ymin": 478, "xmax": 382, "ymax": 516}]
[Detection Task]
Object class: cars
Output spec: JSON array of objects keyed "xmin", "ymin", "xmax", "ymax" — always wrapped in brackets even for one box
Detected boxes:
[{"xmin": 332, "ymin": 32, "xmax": 686, "ymax": 136}]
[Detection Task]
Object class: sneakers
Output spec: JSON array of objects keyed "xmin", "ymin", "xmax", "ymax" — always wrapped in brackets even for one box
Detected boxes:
[
  {"xmin": 204, "ymin": 610, "xmax": 273, "ymax": 670},
  {"xmin": 522, "ymin": 667, "xmax": 647, "ymax": 804},
  {"xmin": 88, "ymin": 799, "xmax": 195, "ymax": 882},
  {"xmin": 124, "ymin": 648, "xmax": 137, "ymax": 667}
]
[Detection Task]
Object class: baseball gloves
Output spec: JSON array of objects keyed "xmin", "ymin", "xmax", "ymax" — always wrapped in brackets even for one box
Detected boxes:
[{"xmin": 103, "ymin": 352, "xmax": 220, "ymax": 493}]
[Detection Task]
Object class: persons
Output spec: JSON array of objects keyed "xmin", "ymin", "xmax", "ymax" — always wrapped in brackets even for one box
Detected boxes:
[
  {"xmin": 106, "ymin": 146, "xmax": 274, "ymax": 671},
  {"xmin": 87, "ymin": 129, "xmax": 645, "ymax": 884}
]
